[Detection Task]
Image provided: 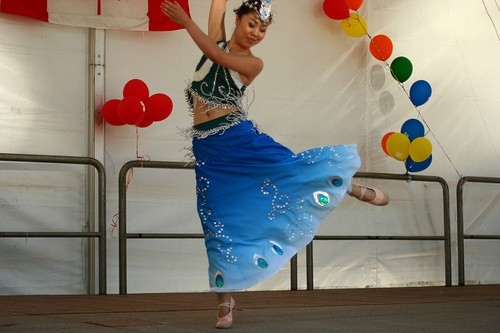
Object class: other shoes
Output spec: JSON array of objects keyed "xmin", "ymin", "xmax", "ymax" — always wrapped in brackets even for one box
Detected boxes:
[
  {"xmin": 352, "ymin": 183, "xmax": 390, "ymax": 206},
  {"xmin": 216, "ymin": 297, "xmax": 236, "ymax": 328}
]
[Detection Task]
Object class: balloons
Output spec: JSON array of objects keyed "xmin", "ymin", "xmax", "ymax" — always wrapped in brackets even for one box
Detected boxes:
[
  {"xmin": 146, "ymin": 94, "xmax": 174, "ymax": 124},
  {"xmin": 322, "ymin": 0, "xmax": 350, "ymax": 21},
  {"xmin": 408, "ymin": 137, "xmax": 433, "ymax": 163},
  {"xmin": 391, "ymin": 56, "xmax": 413, "ymax": 83},
  {"xmin": 410, "ymin": 79, "xmax": 432, "ymax": 107},
  {"xmin": 137, "ymin": 116, "xmax": 153, "ymax": 128},
  {"xmin": 385, "ymin": 133, "xmax": 411, "ymax": 162},
  {"xmin": 380, "ymin": 131, "xmax": 398, "ymax": 158},
  {"xmin": 401, "ymin": 118, "xmax": 425, "ymax": 143},
  {"xmin": 341, "ymin": 12, "xmax": 368, "ymax": 38},
  {"xmin": 369, "ymin": 35, "xmax": 393, "ymax": 62},
  {"xmin": 102, "ymin": 99, "xmax": 127, "ymax": 125},
  {"xmin": 404, "ymin": 152, "xmax": 433, "ymax": 173},
  {"xmin": 122, "ymin": 79, "xmax": 149, "ymax": 101},
  {"xmin": 346, "ymin": 1, "xmax": 364, "ymax": 10},
  {"xmin": 117, "ymin": 96, "xmax": 146, "ymax": 125}
]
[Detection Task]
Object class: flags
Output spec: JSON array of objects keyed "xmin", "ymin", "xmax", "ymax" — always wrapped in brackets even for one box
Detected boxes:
[{"xmin": 1, "ymin": 0, "xmax": 190, "ymax": 33}]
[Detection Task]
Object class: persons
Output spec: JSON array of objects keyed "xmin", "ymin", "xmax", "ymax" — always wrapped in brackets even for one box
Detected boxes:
[{"xmin": 159, "ymin": 0, "xmax": 391, "ymax": 330}]
